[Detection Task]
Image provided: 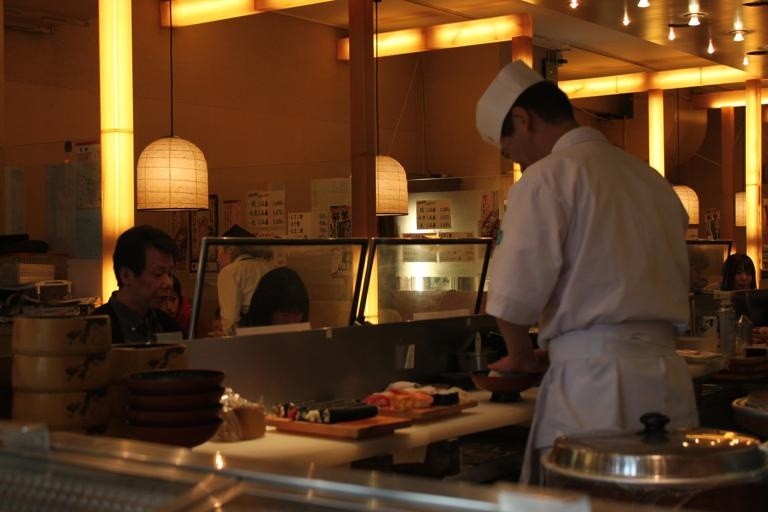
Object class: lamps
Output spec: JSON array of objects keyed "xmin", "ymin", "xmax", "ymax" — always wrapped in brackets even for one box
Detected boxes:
[
  {"xmin": 670, "ymin": 89, "xmax": 700, "ymax": 226},
  {"xmin": 137, "ymin": 1, "xmax": 210, "ymax": 212},
  {"xmin": 373, "ymin": 0, "xmax": 408, "ymax": 217}
]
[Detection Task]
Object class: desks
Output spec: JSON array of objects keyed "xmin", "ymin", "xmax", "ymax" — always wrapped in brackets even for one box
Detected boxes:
[{"xmin": 190, "ymin": 380, "xmax": 535, "ymax": 475}]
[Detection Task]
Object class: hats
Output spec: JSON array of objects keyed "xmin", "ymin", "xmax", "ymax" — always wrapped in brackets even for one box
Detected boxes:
[{"xmin": 475, "ymin": 60, "xmax": 546, "ymax": 150}]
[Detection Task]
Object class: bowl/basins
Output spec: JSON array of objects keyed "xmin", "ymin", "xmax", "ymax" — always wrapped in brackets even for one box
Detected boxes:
[{"xmin": 121, "ymin": 368, "xmax": 227, "ymax": 448}]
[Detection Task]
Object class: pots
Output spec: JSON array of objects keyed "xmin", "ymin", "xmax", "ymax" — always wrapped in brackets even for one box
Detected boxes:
[
  {"xmin": 541, "ymin": 410, "xmax": 768, "ymax": 512},
  {"xmin": 731, "ymin": 392, "xmax": 768, "ymax": 437}
]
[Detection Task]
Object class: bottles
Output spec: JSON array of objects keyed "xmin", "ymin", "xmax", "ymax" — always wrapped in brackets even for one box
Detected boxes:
[{"xmin": 736, "ymin": 313, "xmax": 753, "ymax": 345}]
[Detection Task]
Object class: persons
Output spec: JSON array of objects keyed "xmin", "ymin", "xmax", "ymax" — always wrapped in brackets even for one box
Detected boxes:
[
  {"xmin": 163, "ymin": 277, "xmax": 207, "ymax": 340},
  {"xmin": 86, "ymin": 224, "xmax": 187, "ymax": 342},
  {"xmin": 229, "ymin": 266, "xmax": 309, "ymax": 334},
  {"xmin": 477, "ymin": 57, "xmax": 700, "ymax": 495},
  {"xmin": 209, "ymin": 223, "xmax": 269, "ymax": 338},
  {"xmin": 704, "ymin": 253, "xmax": 759, "ymax": 292}
]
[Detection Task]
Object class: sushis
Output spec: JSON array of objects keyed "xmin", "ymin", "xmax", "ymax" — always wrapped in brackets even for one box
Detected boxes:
[{"xmin": 271, "ymin": 381, "xmax": 459, "ymax": 425}]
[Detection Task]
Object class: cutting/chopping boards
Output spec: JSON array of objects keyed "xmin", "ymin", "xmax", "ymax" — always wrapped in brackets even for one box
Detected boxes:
[
  {"xmin": 263, "ymin": 411, "xmax": 413, "ymax": 439},
  {"xmin": 371, "ymin": 400, "xmax": 478, "ymax": 420}
]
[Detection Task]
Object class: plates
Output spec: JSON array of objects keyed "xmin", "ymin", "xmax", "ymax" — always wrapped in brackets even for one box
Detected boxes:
[{"xmin": 674, "ymin": 348, "xmax": 723, "ymax": 363}]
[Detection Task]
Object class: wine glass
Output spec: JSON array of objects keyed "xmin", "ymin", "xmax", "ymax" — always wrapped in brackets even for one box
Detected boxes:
[{"xmin": 470, "ymin": 368, "xmax": 543, "ymax": 402}]
